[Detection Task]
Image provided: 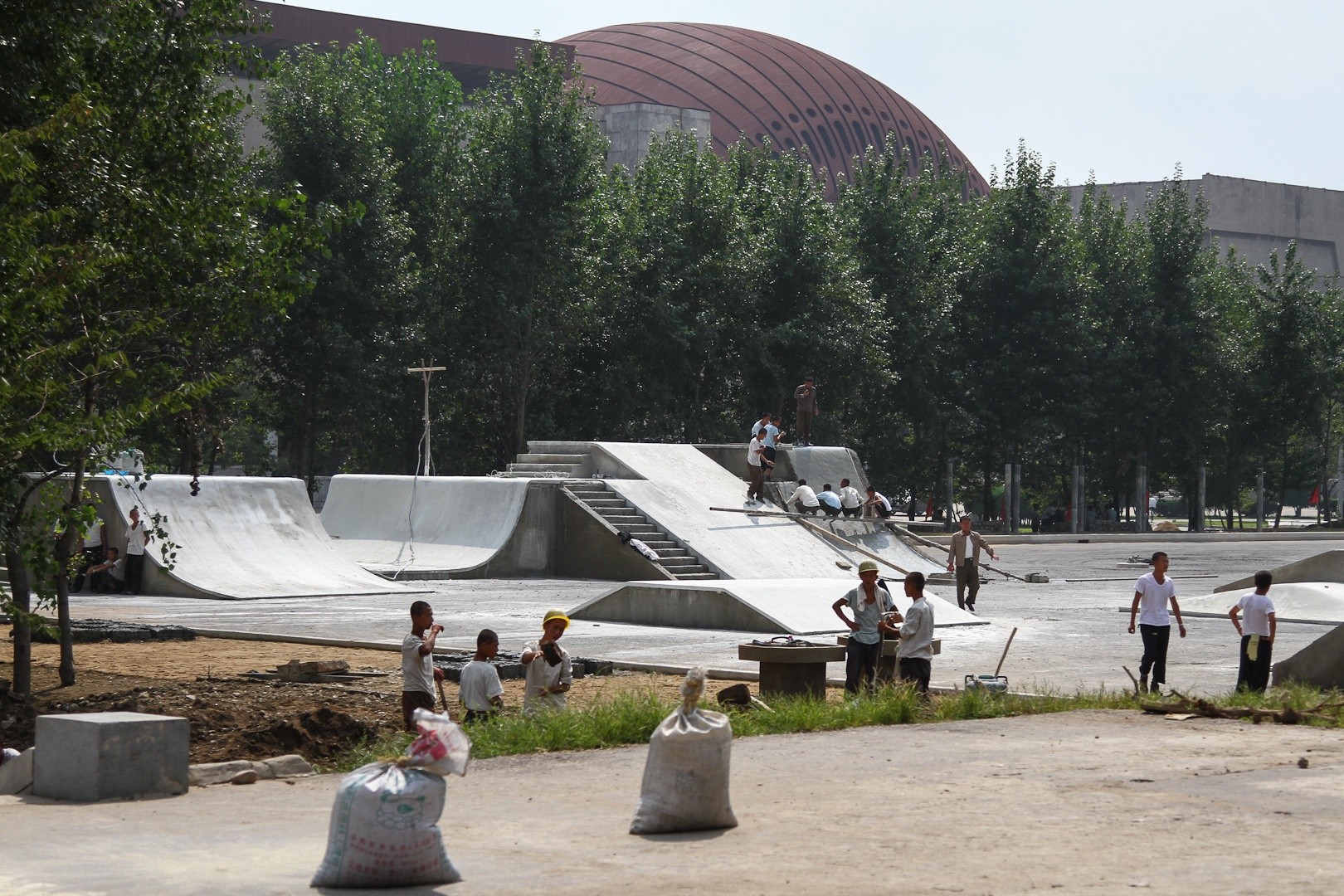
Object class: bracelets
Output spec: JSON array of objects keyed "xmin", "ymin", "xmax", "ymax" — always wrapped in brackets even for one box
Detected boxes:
[
  {"xmin": 1179, "ymin": 624, "xmax": 1184, "ymax": 627},
  {"xmin": 1237, "ymin": 626, "xmax": 1242, "ymax": 630},
  {"xmin": 93, "ymin": 569, "xmax": 95, "ymax": 573}
]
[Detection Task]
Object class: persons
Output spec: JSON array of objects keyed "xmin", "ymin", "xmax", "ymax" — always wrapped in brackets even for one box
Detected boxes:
[
  {"xmin": 864, "ymin": 486, "xmax": 892, "ymax": 519},
  {"xmin": 838, "ymin": 478, "xmax": 864, "ymax": 518},
  {"xmin": 1030, "ymin": 499, "xmax": 1116, "ymax": 533},
  {"xmin": 457, "ymin": 629, "xmax": 504, "ymax": 731},
  {"xmin": 519, "ymin": 611, "xmax": 573, "ymax": 718},
  {"xmin": 1228, "ymin": 570, "xmax": 1276, "ymax": 695},
  {"xmin": 946, "ymin": 516, "xmax": 1000, "ymax": 612},
  {"xmin": 52, "ymin": 501, "xmax": 150, "ymax": 595},
  {"xmin": 877, "ymin": 572, "xmax": 934, "ymax": 698},
  {"xmin": 746, "ymin": 413, "xmax": 787, "ymax": 504},
  {"xmin": 793, "ymin": 376, "xmax": 819, "ymax": 446},
  {"xmin": 816, "ymin": 483, "xmax": 843, "ymax": 521},
  {"xmin": 1127, "ymin": 552, "xmax": 1186, "ymax": 695},
  {"xmin": 786, "ymin": 479, "xmax": 821, "ymax": 516},
  {"xmin": 400, "ymin": 601, "xmax": 443, "ymax": 732},
  {"xmin": 832, "ymin": 561, "xmax": 904, "ymax": 699}
]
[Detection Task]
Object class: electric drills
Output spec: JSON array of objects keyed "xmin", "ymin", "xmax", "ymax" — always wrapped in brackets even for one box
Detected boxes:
[{"xmin": 592, "ymin": 469, "xmax": 612, "ymax": 478}]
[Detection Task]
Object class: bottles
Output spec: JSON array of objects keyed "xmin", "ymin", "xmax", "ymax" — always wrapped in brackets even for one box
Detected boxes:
[{"xmin": 592, "ymin": 473, "xmax": 596, "ymax": 478}]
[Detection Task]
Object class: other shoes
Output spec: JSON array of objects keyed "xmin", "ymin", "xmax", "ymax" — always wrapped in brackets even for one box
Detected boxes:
[
  {"xmin": 747, "ymin": 492, "xmax": 754, "ymax": 499},
  {"xmin": 766, "ymin": 476, "xmax": 771, "ymax": 482},
  {"xmin": 1140, "ymin": 675, "xmax": 1148, "ymax": 693},
  {"xmin": 843, "ymin": 515, "xmax": 850, "ymax": 522},
  {"xmin": 823, "ymin": 513, "xmax": 831, "ymax": 521},
  {"xmin": 812, "ymin": 512, "xmax": 817, "ymax": 518},
  {"xmin": 756, "ymin": 496, "xmax": 766, "ymax": 503},
  {"xmin": 1150, "ymin": 683, "xmax": 1163, "ymax": 696},
  {"xmin": 854, "ymin": 512, "xmax": 862, "ymax": 519},
  {"xmin": 965, "ymin": 600, "xmax": 976, "ymax": 613},
  {"xmin": 797, "ymin": 442, "xmax": 804, "ymax": 447},
  {"xmin": 832, "ymin": 514, "xmax": 838, "ymax": 519},
  {"xmin": 804, "ymin": 442, "xmax": 813, "ymax": 446}
]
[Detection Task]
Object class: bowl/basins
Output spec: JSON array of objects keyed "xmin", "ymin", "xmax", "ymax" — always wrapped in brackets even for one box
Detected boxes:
[
  {"xmin": 104, "ymin": 469, "xmax": 116, "ymax": 474},
  {"xmin": 117, "ymin": 470, "xmax": 130, "ymax": 475},
  {"xmin": 596, "ymin": 474, "xmax": 604, "ymax": 478}
]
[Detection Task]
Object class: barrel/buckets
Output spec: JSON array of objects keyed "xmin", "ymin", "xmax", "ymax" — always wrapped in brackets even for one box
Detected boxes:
[{"xmin": 963, "ymin": 673, "xmax": 1008, "ymax": 697}]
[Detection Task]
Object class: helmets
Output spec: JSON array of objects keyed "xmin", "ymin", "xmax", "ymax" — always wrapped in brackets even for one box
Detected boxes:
[
  {"xmin": 858, "ymin": 561, "xmax": 879, "ymax": 574},
  {"xmin": 542, "ymin": 608, "xmax": 571, "ymax": 631}
]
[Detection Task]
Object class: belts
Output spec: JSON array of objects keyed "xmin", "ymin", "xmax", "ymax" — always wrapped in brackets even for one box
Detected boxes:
[{"xmin": 965, "ymin": 558, "xmax": 973, "ymax": 561}]
